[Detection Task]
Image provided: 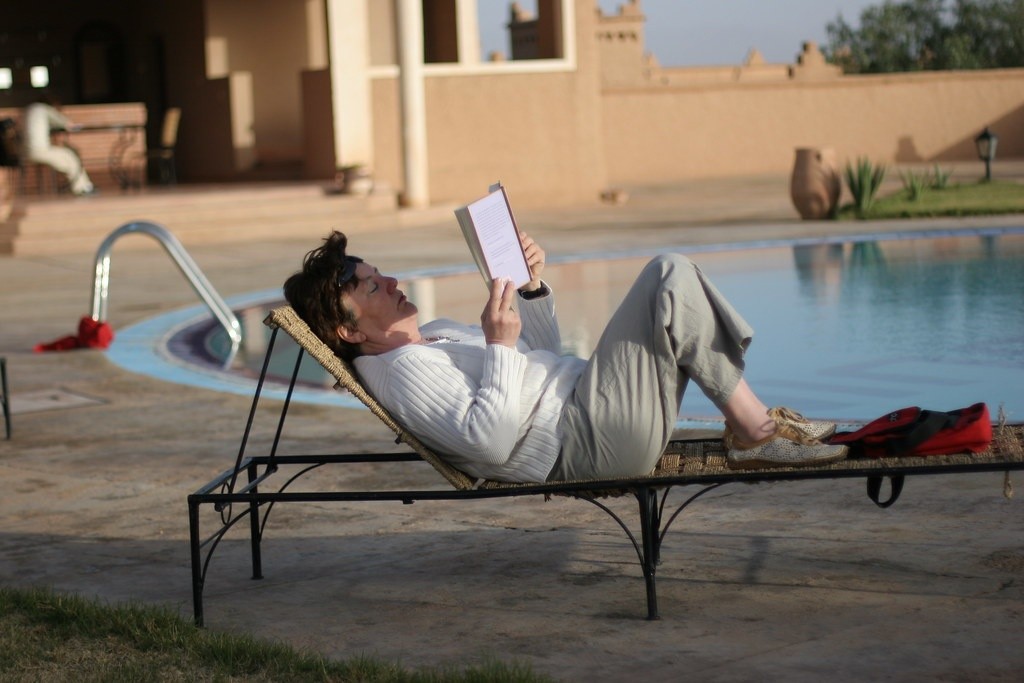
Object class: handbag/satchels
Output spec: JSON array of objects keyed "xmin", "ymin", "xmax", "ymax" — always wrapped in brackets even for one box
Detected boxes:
[{"xmin": 824, "ymin": 404, "xmax": 992, "ymax": 508}]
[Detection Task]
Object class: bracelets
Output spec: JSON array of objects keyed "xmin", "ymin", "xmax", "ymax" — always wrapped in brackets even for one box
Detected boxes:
[{"xmin": 517, "ymin": 282, "xmax": 546, "ymax": 300}]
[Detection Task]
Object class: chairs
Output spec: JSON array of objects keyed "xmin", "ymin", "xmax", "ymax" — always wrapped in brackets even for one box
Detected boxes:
[
  {"xmin": 120, "ymin": 103, "xmax": 184, "ymax": 194},
  {"xmin": 0, "ymin": 116, "xmax": 58, "ymax": 195},
  {"xmin": 188, "ymin": 301, "xmax": 1023, "ymax": 630}
]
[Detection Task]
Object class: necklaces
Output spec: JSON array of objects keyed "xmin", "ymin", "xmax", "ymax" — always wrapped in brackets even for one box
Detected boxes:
[{"xmin": 424, "ymin": 337, "xmax": 460, "ymax": 342}]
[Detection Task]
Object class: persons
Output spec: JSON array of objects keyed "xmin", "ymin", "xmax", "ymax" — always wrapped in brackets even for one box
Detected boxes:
[
  {"xmin": 20, "ymin": 101, "xmax": 94, "ymax": 196},
  {"xmin": 287, "ymin": 230, "xmax": 850, "ymax": 484}
]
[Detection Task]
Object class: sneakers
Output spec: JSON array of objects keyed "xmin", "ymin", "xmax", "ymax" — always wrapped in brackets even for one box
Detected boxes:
[
  {"xmin": 722, "ymin": 406, "xmax": 835, "ymax": 447},
  {"xmin": 727, "ymin": 418, "xmax": 847, "ymax": 469}
]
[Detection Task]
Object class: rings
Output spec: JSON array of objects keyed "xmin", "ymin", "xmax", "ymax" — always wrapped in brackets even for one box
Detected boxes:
[{"xmin": 510, "ymin": 305, "xmax": 515, "ymax": 312}]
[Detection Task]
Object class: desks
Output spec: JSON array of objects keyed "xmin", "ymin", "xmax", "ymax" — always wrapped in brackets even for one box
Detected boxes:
[{"xmin": 49, "ymin": 121, "xmax": 151, "ymax": 192}]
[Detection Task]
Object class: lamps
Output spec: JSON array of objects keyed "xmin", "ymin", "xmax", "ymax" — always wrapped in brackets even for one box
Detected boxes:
[{"xmin": 973, "ymin": 126, "xmax": 1000, "ymax": 162}]
